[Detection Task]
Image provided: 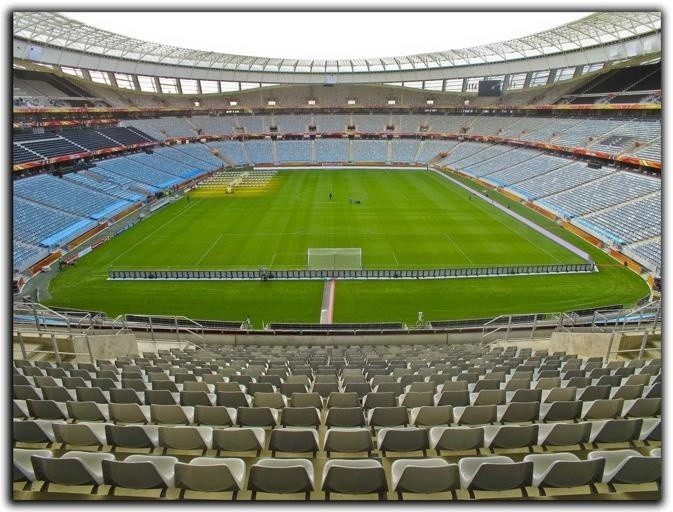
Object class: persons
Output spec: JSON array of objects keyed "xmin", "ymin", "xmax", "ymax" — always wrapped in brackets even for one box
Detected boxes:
[
  {"xmin": 56, "ymin": 256, "xmax": 74, "ymax": 272},
  {"xmin": 143, "ymin": 182, "xmax": 179, "ymax": 206}
]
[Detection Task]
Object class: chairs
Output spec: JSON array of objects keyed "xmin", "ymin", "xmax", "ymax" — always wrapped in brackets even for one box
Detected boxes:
[
  {"xmin": 500, "ymin": 118, "xmax": 662, "ymax": 274},
  {"xmin": 13, "ymin": 112, "xmax": 228, "ymax": 273},
  {"xmin": 12, "ymin": 341, "xmax": 661, "ymax": 503}
]
